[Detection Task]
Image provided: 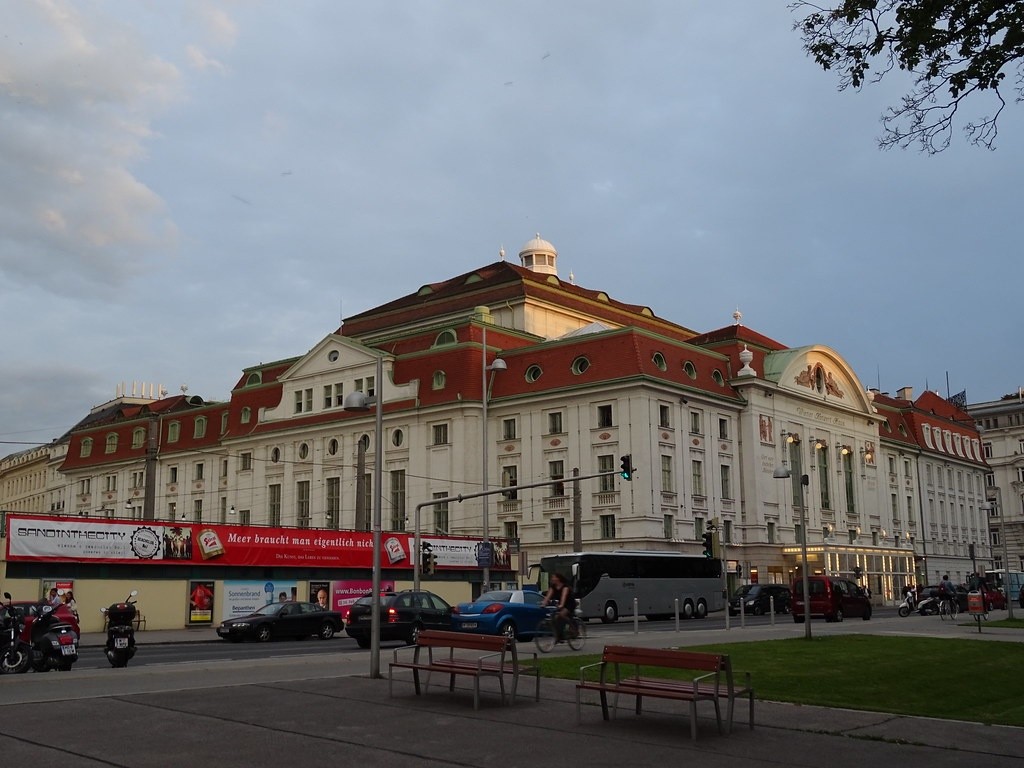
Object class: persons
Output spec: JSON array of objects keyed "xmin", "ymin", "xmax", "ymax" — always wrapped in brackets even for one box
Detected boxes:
[
  {"xmin": 189, "ymin": 584, "xmax": 213, "ymax": 610},
  {"xmin": 543, "ymin": 573, "xmax": 578, "ymax": 639},
  {"xmin": 315, "ymin": 590, "xmax": 329, "ymax": 611},
  {"xmin": 64, "ymin": 591, "xmax": 77, "ymax": 614},
  {"xmin": 939, "ymin": 575, "xmax": 957, "ymax": 610},
  {"xmin": 279, "ymin": 592, "xmax": 286, "ymax": 602},
  {"xmin": 51, "ymin": 588, "xmax": 61, "ymax": 604}
]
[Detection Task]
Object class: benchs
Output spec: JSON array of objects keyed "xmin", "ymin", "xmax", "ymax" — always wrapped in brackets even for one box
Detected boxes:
[
  {"xmin": 388, "ymin": 630, "xmax": 541, "ymax": 712},
  {"xmin": 575, "ymin": 645, "xmax": 754, "ymax": 741}
]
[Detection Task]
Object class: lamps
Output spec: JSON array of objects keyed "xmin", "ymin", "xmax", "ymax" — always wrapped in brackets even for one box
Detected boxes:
[
  {"xmin": 325, "ymin": 511, "xmax": 332, "ymax": 520},
  {"xmin": 836, "ymin": 445, "xmax": 848, "ymax": 455},
  {"xmin": 77, "ymin": 509, "xmax": 84, "ymax": 519},
  {"xmin": 810, "ymin": 438, "xmax": 822, "ymax": 449},
  {"xmin": 229, "ymin": 505, "xmax": 236, "ymax": 515},
  {"xmin": 780, "ymin": 432, "xmax": 793, "ymax": 443},
  {"xmin": 181, "ymin": 513, "xmax": 187, "ymax": 521},
  {"xmin": 125, "ymin": 500, "xmax": 132, "ymax": 509},
  {"xmin": 860, "ymin": 450, "xmax": 872, "ymax": 460}
]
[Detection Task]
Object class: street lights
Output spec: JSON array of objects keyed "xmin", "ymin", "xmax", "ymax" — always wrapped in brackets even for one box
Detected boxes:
[
  {"xmin": 481, "ymin": 359, "xmax": 506, "ymax": 591},
  {"xmin": 773, "ymin": 467, "xmax": 812, "ymax": 637},
  {"xmin": 979, "ymin": 502, "xmax": 1013, "ymax": 619},
  {"xmin": 344, "ymin": 392, "xmax": 381, "ymax": 677}
]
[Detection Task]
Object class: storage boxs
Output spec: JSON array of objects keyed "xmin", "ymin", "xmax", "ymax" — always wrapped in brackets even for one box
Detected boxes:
[{"xmin": 108, "ymin": 603, "xmax": 136, "ymax": 623}]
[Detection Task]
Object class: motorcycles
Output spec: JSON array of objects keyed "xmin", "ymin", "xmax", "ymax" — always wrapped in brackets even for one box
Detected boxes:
[{"xmin": 26, "ymin": 598, "xmax": 78, "ymax": 672}]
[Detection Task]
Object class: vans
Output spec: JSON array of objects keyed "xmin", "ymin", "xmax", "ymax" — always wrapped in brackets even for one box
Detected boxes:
[{"xmin": 791, "ymin": 576, "xmax": 872, "ymax": 622}]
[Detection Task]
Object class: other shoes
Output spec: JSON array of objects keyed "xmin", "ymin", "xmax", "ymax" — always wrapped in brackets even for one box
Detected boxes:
[{"xmin": 573, "ymin": 620, "xmax": 580, "ymax": 632}]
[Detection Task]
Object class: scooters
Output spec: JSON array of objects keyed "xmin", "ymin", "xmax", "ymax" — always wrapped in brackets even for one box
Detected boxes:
[
  {"xmin": 103, "ymin": 590, "xmax": 138, "ymax": 669},
  {"xmin": 898, "ymin": 590, "xmax": 935, "ymax": 617}
]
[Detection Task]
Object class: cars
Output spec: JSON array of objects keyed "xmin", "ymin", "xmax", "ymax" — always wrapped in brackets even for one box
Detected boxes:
[
  {"xmin": 982, "ymin": 584, "xmax": 1008, "ymax": 611},
  {"xmin": 216, "ymin": 600, "xmax": 343, "ymax": 642},
  {"xmin": 0, "ymin": 600, "xmax": 80, "ymax": 647},
  {"xmin": 919, "ymin": 585, "xmax": 968, "ymax": 612},
  {"xmin": 728, "ymin": 584, "xmax": 794, "ymax": 615},
  {"xmin": 452, "ymin": 591, "xmax": 558, "ymax": 642}
]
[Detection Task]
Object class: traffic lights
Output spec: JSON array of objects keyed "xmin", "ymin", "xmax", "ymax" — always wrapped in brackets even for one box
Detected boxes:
[
  {"xmin": 422, "ymin": 553, "xmax": 438, "ymax": 576},
  {"xmin": 701, "ymin": 533, "xmax": 713, "ymax": 558},
  {"xmin": 422, "ymin": 541, "xmax": 431, "ymax": 551},
  {"xmin": 620, "ymin": 454, "xmax": 632, "ymax": 480}
]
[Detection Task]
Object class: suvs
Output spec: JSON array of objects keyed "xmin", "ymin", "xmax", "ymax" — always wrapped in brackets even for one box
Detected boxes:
[{"xmin": 345, "ymin": 588, "xmax": 456, "ymax": 648}]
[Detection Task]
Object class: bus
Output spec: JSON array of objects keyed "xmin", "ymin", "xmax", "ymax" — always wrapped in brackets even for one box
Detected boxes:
[
  {"xmin": 526, "ymin": 550, "xmax": 725, "ymax": 624},
  {"xmin": 967, "ymin": 569, "xmax": 1024, "ymax": 600}
]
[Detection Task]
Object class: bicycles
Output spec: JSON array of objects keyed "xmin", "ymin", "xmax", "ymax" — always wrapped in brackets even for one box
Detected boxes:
[
  {"xmin": 973, "ymin": 593, "xmax": 989, "ymax": 621},
  {"xmin": 534, "ymin": 605, "xmax": 587, "ymax": 654},
  {"xmin": 939, "ymin": 595, "xmax": 958, "ymax": 621}
]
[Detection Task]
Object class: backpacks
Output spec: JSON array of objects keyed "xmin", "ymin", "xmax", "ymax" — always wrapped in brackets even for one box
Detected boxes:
[{"xmin": 939, "ymin": 581, "xmax": 945, "ymax": 593}]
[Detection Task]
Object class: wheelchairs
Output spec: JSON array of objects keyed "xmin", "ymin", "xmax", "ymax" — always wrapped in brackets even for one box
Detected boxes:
[{"xmin": 0, "ymin": 592, "xmax": 33, "ymax": 674}]
